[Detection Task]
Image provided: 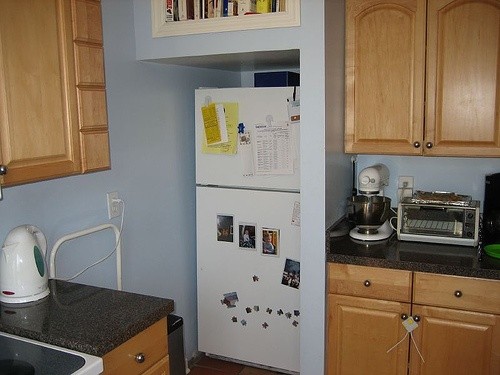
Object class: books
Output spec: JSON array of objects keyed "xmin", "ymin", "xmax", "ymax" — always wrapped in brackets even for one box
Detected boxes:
[{"xmin": 165, "ymin": 0, "xmax": 285, "ymax": 22}]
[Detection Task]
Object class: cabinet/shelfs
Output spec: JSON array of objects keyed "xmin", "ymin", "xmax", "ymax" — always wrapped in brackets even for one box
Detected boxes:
[
  {"xmin": 101, "ymin": 316, "xmax": 169, "ymax": 374},
  {"xmin": 1, "ymin": 1, "xmax": 82, "ymax": 188},
  {"xmin": 344, "ymin": 0, "xmax": 500, "ymax": 158},
  {"xmin": 324, "ymin": 262, "xmax": 499, "ymax": 375}
]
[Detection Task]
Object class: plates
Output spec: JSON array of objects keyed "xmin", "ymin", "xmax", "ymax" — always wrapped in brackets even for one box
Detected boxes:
[{"xmin": 484, "ymin": 245, "xmax": 499, "ymax": 259}]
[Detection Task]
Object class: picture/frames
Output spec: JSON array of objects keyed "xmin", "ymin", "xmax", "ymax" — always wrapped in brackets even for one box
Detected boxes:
[{"xmin": 151, "ymin": 1, "xmax": 301, "ymax": 39}]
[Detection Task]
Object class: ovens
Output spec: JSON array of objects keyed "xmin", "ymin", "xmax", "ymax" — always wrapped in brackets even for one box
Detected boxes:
[{"xmin": 398, "ymin": 197, "xmax": 480, "ymax": 246}]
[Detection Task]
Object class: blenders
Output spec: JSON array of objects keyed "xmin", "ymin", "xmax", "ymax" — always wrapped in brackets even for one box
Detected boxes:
[{"xmin": 347, "ymin": 164, "xmax": 393, "ymax": 241}]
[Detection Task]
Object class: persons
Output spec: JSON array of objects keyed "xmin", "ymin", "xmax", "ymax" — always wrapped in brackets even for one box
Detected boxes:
[
  {"xmin": 264, "ymin": 233, "xmax": 275, "ymax": 253},
  {"xmin": 286, "ymin": 271, "xmax": 296, "ymax": 286},
  {"xmin": 243, "ymin": 230, "xmax": 253, "ymax": 248}
]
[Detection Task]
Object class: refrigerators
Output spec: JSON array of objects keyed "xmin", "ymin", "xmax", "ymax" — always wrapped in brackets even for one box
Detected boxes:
[{"xmin": 194, "ymin": 87, "xmax": 301, "ymax": 374}]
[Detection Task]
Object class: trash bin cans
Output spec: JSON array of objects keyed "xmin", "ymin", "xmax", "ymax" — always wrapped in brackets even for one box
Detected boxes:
[{"xmin": 168, "ymin": 315, "xmax": 185, "ymax": 375}]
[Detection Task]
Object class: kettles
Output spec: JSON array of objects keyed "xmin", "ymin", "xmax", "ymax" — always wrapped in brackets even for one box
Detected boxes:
[{"xmin": 1, "ymin": 225, "xmax": 53, "ymax": 305}]
